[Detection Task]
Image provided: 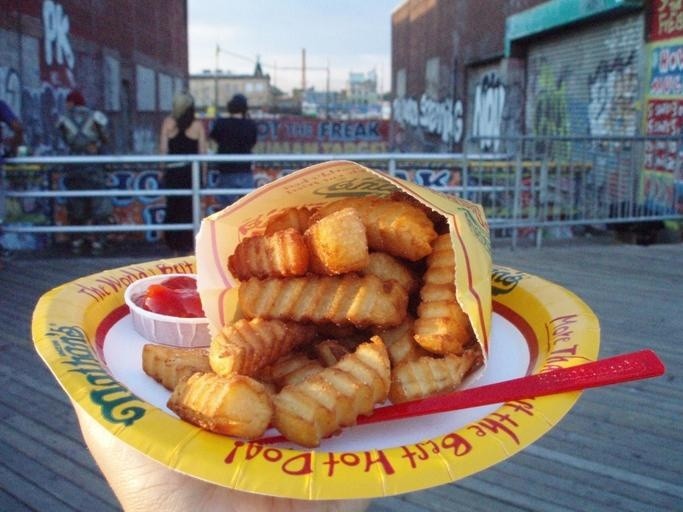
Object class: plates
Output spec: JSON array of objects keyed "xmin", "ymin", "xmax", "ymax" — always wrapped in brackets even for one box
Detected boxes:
[{"xmin": 31, "ymin": 257, "xmax": 602, "ymax": 500}]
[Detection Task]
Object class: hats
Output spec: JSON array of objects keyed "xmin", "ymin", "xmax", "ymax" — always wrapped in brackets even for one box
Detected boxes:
[{"xmin": 67, "ymin": 90, "xmax": 85, "ymax": 105}]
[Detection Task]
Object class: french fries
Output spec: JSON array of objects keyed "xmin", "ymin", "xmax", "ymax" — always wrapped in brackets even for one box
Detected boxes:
[{"xmin": 142, "ymin": 189, "xmax": 475, "ymax": 446}]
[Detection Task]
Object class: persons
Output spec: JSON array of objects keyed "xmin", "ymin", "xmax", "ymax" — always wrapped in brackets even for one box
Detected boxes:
[
  {"xmin": 54, "ymin": 89, "xmax": 116, "ymax": 256},
  {"xmin": 209, "ymin": 94, "xmax": 257, "ymax": 208},
  {"xmin": 156, "ymin": 91, "xmax": 206, "ymax": 255},
  {"xmin": 0, "ymin": 98, "xmax": 24, "ymax": 148}
]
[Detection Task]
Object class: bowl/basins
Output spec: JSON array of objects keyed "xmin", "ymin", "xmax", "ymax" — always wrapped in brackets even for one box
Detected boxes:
[{"xmin": 125, "ymin": 272, "xmax": 206, "ymax": 345}]
